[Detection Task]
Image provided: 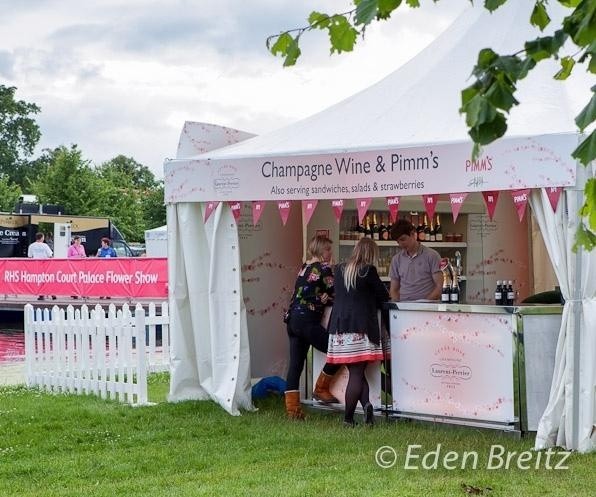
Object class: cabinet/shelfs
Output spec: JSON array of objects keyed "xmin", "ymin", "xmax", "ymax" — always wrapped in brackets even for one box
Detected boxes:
[{"xmin": 338, "ymin": 238, "xmax": 468, "ymax": 283}]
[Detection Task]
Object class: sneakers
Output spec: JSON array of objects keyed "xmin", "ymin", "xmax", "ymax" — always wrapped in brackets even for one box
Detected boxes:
[
  {"xmin": 363, "ymin": 403, "xmax": 374, "ymax": 423},
  {"xmin": 343, "ymin": 420, "xmax": 359, "ymax": 428}
]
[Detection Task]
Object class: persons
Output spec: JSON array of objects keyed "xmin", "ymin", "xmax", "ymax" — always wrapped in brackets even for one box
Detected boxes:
[
  {"xmin": 43, "ymin": 232, "xmax": 53, "ymax": 251},
  {"xmin": 325, "ymin": 236, "xmax": 391, "ymax": 427},
  {"xmin": 95, "ymin": 237, "xmax": 117, "ymax": 257},
  {"xmin": 387, "ymin": 218, "xmax": 444, "ymax": 302},
  {"xmin": 28, "ymin": 232, "xmax": 52, "ymax": 258},
  {"xmin": 281, "ymin": 234, "xmax": 341, "ymax": 421},
  {"xmin": 67, "ymin": 234, "xmax": 86, "ymax": 258}
]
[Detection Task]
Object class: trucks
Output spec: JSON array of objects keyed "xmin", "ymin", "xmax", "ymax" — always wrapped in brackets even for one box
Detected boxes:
[{"xmin": 0, "ymin": 210, "xmax": 134, "ymax": 258}]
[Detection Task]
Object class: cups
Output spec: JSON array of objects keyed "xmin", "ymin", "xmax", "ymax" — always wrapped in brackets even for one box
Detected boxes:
[
  {"xmin": 98, "ymin": 248, "xmax": 101, "ymax": 254},
  {"xmin": 445, "ymin": 232, "xmax": 463, "ymax": 241}
]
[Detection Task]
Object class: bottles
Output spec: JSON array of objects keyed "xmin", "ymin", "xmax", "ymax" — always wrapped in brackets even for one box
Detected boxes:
[
  {"xmin": 342, "ymin": 213, "xmax": 443, "ymax": 241},
  {"xmin": 441, "ymin": 270, "xmax": 450, "ymax": 303},
  {"xmin": 455, "ymin": 250, "xmax": 462, "ymax": 275},
  {"xmin": 451, "ymin": 270, "xmax": 461, "ymax": 303},
  {"xmin": 377, "ymin": 247, "xmax": 400, "ymax": 276},
  {"xmin": 496, "ymin": 280, "xmax": 516, "ymax": 305}
]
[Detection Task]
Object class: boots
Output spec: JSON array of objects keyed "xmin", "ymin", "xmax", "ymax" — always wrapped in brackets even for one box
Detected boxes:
[
  {"xmin": 284, "ymin": 390, "xmax": 304, "ymax": 419},
  {"xmin": 313, "ymin": 371, "xmax": 338, "ymax": 402}
]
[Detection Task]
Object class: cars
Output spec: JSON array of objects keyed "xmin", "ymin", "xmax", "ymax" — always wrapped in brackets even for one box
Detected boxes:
[{"xmin": 111, "ymin": 243, "xmax": 146, "ymax": 257}]
